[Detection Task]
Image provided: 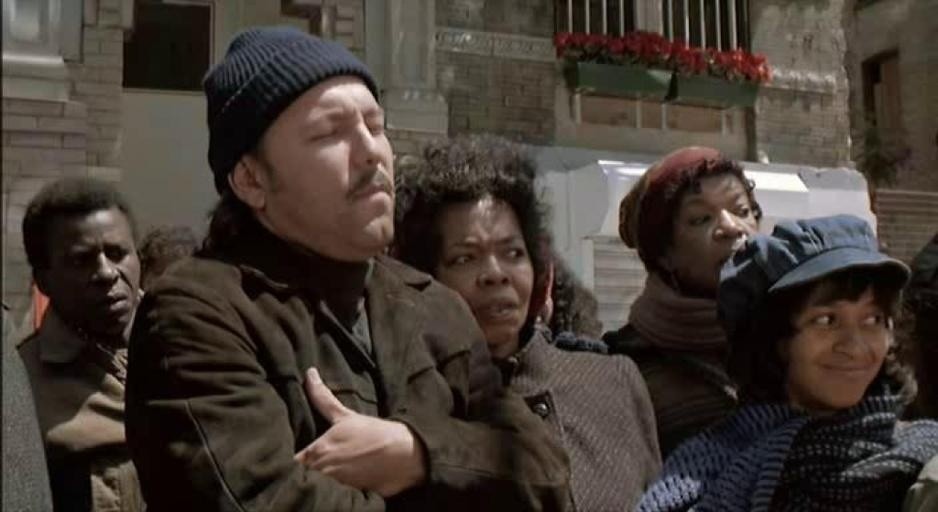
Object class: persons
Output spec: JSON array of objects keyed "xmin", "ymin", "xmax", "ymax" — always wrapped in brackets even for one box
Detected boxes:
[
  {"xmin": 1, "ymin": 178, "xmax": 200, "ymax": 512},
  {"xmin": 123, "ymin": 25, "xmax": 569, "ymax": 512},
  {"xmin": 378, "ymin": 133, "xmax": 665, "ymax": 512},
  {"xmin": 601, "ymin": 145, "xmax": 936, "ymax": 511}
]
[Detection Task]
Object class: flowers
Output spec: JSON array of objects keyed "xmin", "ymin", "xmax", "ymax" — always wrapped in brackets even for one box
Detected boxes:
[{"xmin": 553, "ymin": 28, "xmax": 772, "ymax": 87}]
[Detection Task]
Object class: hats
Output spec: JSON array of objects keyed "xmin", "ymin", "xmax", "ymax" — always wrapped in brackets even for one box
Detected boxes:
[
  {"xmin": 619, "ymin": 146, "xmax": 725, "ymax": 269},
  {"xmin": 718, "ymin": 215, "xmax": 911, "ymax": 341},
  {"xmin": 202, "ymin": 25, "xmax": 378, "ymax": 195}
]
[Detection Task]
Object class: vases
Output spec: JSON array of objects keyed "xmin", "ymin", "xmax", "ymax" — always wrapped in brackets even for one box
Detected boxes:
[{"xmin": 563, "ymin": 61, "xmax": 744, "ymax": 110}]
